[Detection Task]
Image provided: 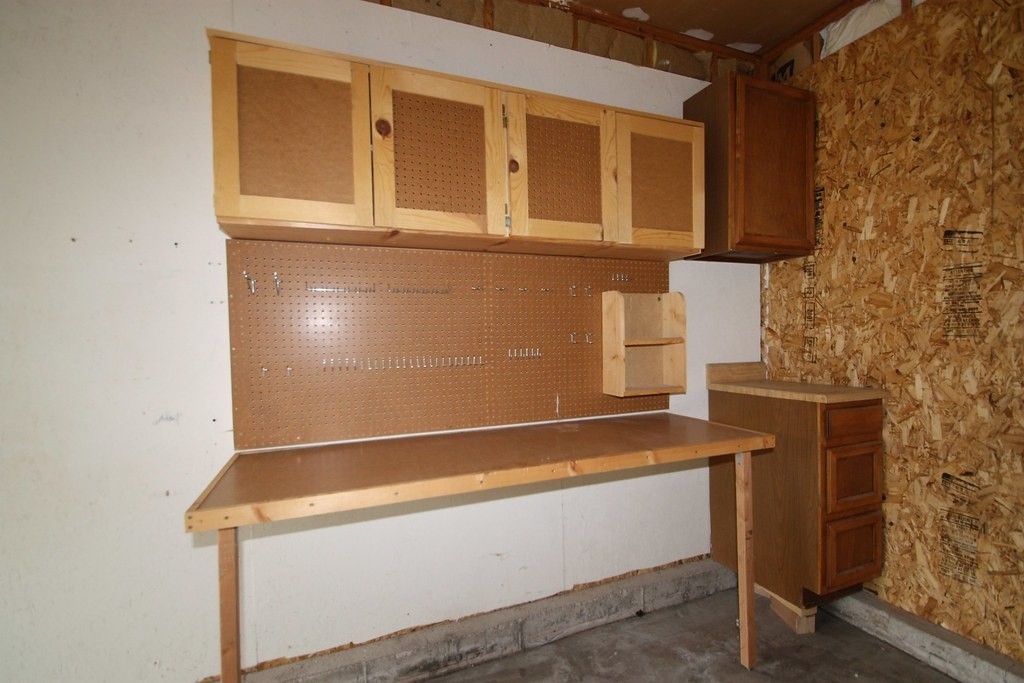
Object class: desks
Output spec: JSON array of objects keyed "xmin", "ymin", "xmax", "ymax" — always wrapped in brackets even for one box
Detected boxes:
[{"xmin": 186, "ymin": 412, "xmax": 775, "ymax": 682}]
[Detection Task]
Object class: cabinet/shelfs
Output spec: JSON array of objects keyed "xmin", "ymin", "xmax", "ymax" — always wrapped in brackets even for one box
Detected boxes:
[
  {"xmin": 683, "ymin": 72, "xmax": 816, "ymax": 265},
  {"xmin": 706, "ymin": 380, "xmax": 884, "ymax": 608},
  {"xmin": 603, "ymin": 290, "xmax": 688, "ymax": 398},
  {"xmin": 205, "ymin": 27, "xmax": 705, "ymax": 262}
]
